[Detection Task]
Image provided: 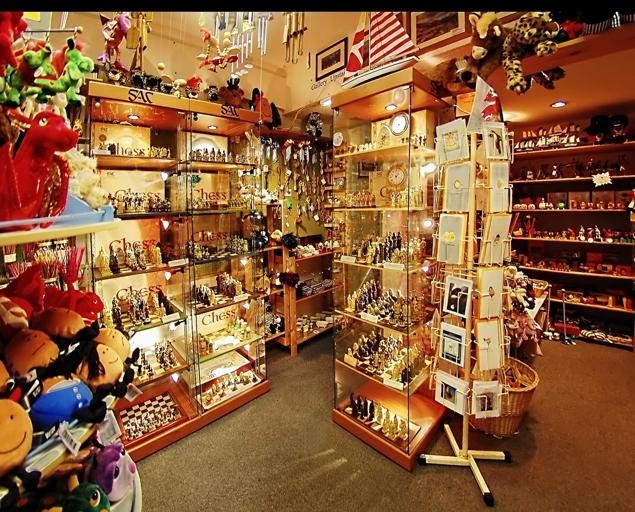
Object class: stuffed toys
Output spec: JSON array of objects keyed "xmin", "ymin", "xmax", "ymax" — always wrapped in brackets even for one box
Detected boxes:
[
  {"xmin": 141, "ymin": 70, "xmax": 274, "ymax": 124},
  {"xmin": 428, "ymin": 11, "xmax": 635, "ymax": 98},
  {"xmin": 306, "ymin": 111, "xmax": 323, "ymax": 136},
  {"xmin": 0, "ymin": 10, "xmax": 140, "ymax": 512}
]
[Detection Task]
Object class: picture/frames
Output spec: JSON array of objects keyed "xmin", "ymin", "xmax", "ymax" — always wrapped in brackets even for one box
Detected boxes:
[
  {"xmin": 314, "ymin": 37, "xmax": 348, "ymax": 82},
  {"xmin": 405, "ymin": 10, "xmax": 473, "ymax": 56}
]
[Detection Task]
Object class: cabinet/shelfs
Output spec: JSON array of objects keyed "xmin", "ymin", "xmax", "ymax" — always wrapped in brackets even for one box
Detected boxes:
[
  {"xmin": 60, "ymin": 67, "xmax": 266, "ymax": 471},
  {"xmin": 264, "ymin": 66, "xmax": 342, "ymax": 472},
  {"xmin": 330, "ymin": 68, "xmax": 549, "ymax": 470},
  {"xmin": 509, "ymin": 109, "xmax": 634, "ymax": 350}
]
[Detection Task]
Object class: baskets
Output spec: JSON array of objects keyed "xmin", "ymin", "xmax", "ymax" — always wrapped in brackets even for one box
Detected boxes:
[{"xmin": 467, "ymin": 355, "xmax": 540, "ymax": 439}]
